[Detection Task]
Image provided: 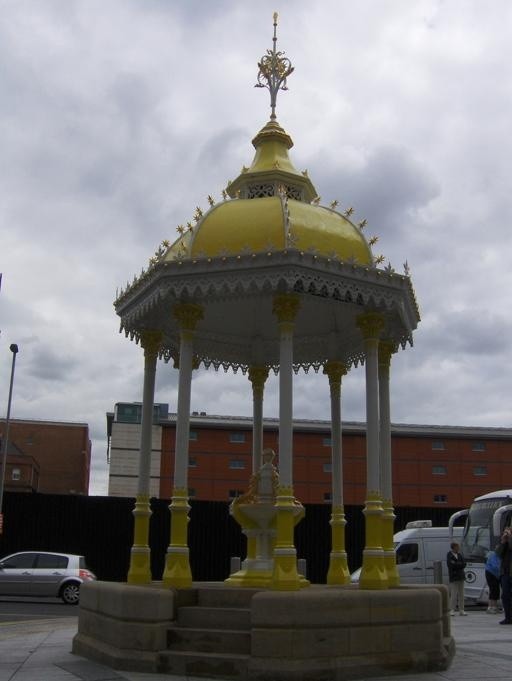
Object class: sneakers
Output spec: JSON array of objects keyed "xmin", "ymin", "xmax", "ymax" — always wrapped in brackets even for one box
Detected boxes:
[
  {"xmin": 486, "ymin": 607, "xmax": 499, "ymax": 614},
  {"xmin": 499, "ymin": 618, "xmax": 512, "ymax": 624},
  {"xmin": 450, "ymin": 610, "xmax": 468, "ymax": 617}
]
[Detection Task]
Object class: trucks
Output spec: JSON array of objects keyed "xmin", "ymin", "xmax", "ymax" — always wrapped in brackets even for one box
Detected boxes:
[{"xmin": 347, "ymin": 523, "xmax": 463, "ymax": 598}]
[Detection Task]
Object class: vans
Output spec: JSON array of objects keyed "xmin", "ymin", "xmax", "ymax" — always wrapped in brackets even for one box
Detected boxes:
[{"xmin": 2, "ymin": 549, "xmax": 99, "ymax": 607}]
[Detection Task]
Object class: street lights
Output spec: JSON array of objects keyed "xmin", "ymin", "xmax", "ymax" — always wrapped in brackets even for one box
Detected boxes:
[{"xmin": 1, "ymin": 341, "xmax": 22, "ymax": 517}]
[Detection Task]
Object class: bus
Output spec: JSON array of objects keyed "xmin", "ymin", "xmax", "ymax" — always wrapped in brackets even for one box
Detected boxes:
[{"xmin": 447, "ymin": 488, "xmax": 512, "ymax": 611}]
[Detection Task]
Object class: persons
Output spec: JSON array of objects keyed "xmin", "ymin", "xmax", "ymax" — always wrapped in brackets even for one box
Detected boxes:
[
  {"xmin": 483, "ymin": 545, "xmax": 503, "ymax": 614},
  {"xmin": 493, "ymin": 526, "xmax": 511, "ymax": 625},
  {"xmin": 445, "ymin": 542, "xmax": 469, "ymax": 618}
]
